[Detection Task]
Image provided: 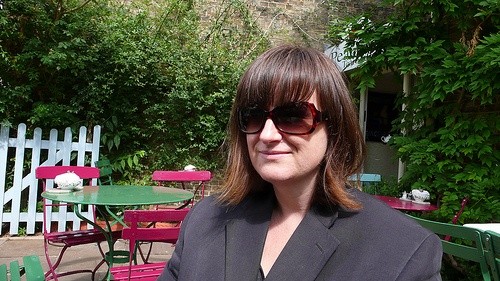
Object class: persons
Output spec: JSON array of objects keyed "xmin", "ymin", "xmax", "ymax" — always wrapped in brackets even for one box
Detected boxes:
[{"xmin": 156, "ymin": 45, "xmax": 444, "ymax": 281}]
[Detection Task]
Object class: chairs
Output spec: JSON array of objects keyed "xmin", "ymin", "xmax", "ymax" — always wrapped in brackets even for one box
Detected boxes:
[
  {"xmin": 35, "ymin": 166, "xmax": 212, "ymax": 281},
  {"xmin": 0, "ymin": 254, "xmax": 45, "ymax": 281},
  {"xmin": 404, "ymin": 199, "xmax": 500, "ymax": 281}
]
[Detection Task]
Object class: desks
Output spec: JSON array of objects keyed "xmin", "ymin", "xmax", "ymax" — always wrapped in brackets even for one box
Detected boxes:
[
  {"xmin": 348, "ymin": 173, "xmax": 382, "ymax": 190},
  {"xmin": 371, "ymin": 195, "xmax": 439, "ymax": 211},
  {"xmin": 41, "ymin": 185, "xmax": 194, "ymax": 281}
]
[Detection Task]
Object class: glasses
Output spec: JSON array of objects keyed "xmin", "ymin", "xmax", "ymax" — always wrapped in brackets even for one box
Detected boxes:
[{"xmin": 236, "ymin": 101, "xmax": 327, "ymax": 135}]
